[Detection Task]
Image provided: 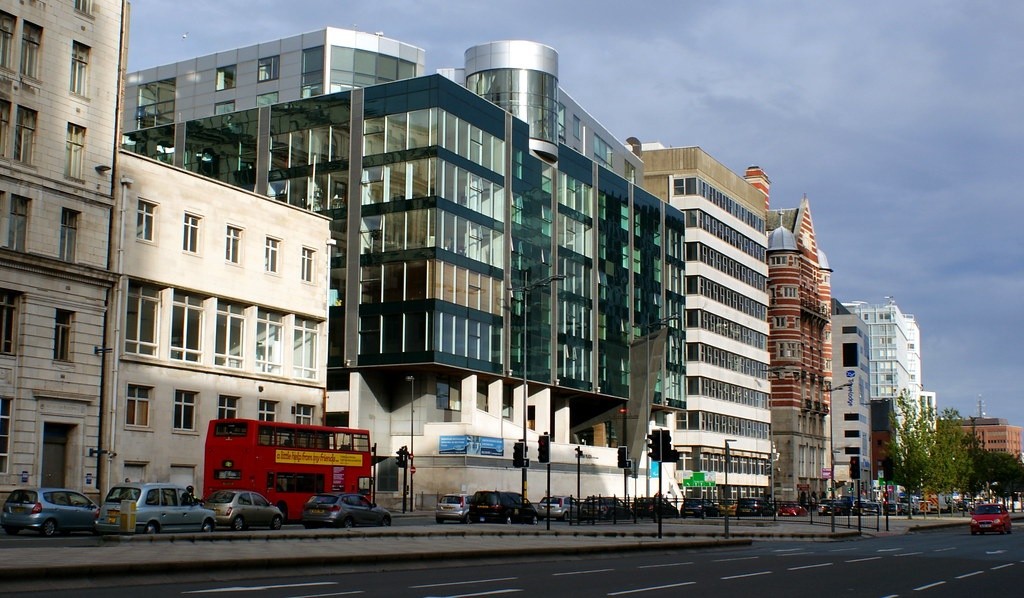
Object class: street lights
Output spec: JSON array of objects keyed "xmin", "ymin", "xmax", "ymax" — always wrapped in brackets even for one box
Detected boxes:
[
  {"xmin": 725, "ymin": 439, "xmax": 738, "ymax": 540},
  {"xmin": 761, "ymin": 365, "xmax": 787, "ymax": 506},
  {"xmin": 633, "ymin": 317, "xmax": 678, "ymax": 497},
  {"xmin": 822, "ymin": 383, "xmax": 854, "ymax": 531},
  {"xmin": 406, "ymin": 375, "xmax": 415, "ymax": 512},
  {"xmin": 522, "ymin": 275, "xmax": 566, "ymax": 522}
]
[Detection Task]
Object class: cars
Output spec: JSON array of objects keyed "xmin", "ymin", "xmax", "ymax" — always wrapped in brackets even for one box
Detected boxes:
[
  {"xmin": 817, "ymin": 495, "xmax": 918, "ymax": 516},
  {"xmin": 94, "ymin": 482, "xmax": 218, "ymax": 535},
  {"xmin": 436, "ymin": 493, "xmax": 474, "ymax": 524},
  {"xmin": 0, "ymin": 488, "xmax": 100, "ymax": 537},
  {"xmin": 719, "ymin": 498, "xmax": 808, "ymax": 516},
  {"xmin": 581, "ymin": 496, "xmax": 721, "ymax": 521},
  {"xmin": 971, "ymin": 503, "xmax": 1012, "ymax": 533},
  {"xmin": 204, "ymin": 490, "xmax": 284, "ymax": 531},
  {"xmin": 537, "ymin": 495, "xmax": 581, "ymax": 521}
]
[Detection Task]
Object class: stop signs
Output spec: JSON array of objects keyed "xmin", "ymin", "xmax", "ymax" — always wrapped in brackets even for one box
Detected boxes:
[{"xmin": 410, "ymin": 467, "xmax": 416, "ymax": 473}]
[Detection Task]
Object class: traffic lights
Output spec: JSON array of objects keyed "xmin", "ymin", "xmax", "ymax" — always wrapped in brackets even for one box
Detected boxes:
[
  {"xmin": 395, "ymin": 449, "xmax": 403, "ymax": 466},
  {"xmin": 849, "ymin": 456, "xmax": 861, "ymax": 480},
  {"xmin": 513, "ymin": 442, "xmax": 525, "ymax": 467},
  {"xmin": 618, "ymin": 446, "xmax": 632, "ymax": 468},
  {"xmin": 647, "ymin": 429, "xmax": 662, "ymax": 462},
  {"xmin": 861, "ymin": 482, "xmax": 864, "ymax": 489},
  {"xmin": 537, "ymin": 435, "xmax": 550, "ymax": 464}
]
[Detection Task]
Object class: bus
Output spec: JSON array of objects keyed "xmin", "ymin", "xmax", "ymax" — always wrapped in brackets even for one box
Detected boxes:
[{"xmin": 203, "ymin": 419, "xmax": 373, "ymax": 523}]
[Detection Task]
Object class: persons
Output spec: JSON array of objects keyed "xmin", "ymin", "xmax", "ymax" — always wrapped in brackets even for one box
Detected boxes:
[
  {"xmin": 119, "ymin": 477, "xmax": 135, "ymax": 499},
  {"xmin": 800, "ymin": 491, "xmax": 806, "ymax": 506},
  {"xmin": 821, "ymin": 491, "xmax": 826, "ymax": 499},
  {"xmin": 666, "ymin": 491, "xmax": 674, "ymax": 505},
  {"xmin": 654, "ymin": 491, "xmax": 664, "ymax": 498},
  {"xmin": 811, "ymin": 491, "xmax": 817, "ymax": 511},
  {"xmin": 147, "ymin": 490, "xmax": 159, "ymax": 505},
  {"xmin": 180, "ymin": 486, "xmax": 205, "ymax": 506},
  {"xmin": 134, "ymin": 480, "xmax": 145, "ymax": 499}
]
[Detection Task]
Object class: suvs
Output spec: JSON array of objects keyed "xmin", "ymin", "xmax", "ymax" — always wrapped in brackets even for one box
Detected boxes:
[{"xmin": 302, "ymin": 493, "xmax": 392, "ymax": 529}]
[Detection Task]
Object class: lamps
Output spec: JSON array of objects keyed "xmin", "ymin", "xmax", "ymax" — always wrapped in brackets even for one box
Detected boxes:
[{"xmin": 94, "ymin": 165, "xmax": 111, "ymax": 171}]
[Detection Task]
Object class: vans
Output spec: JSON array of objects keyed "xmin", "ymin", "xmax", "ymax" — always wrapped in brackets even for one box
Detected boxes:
[
  {"xmin": 924, "ymin": 494, "xmax": 957, "ymax": 511},
  {"xmin": 470, "ymin": 491, "xmax": 539, "ymax": 525}
]
